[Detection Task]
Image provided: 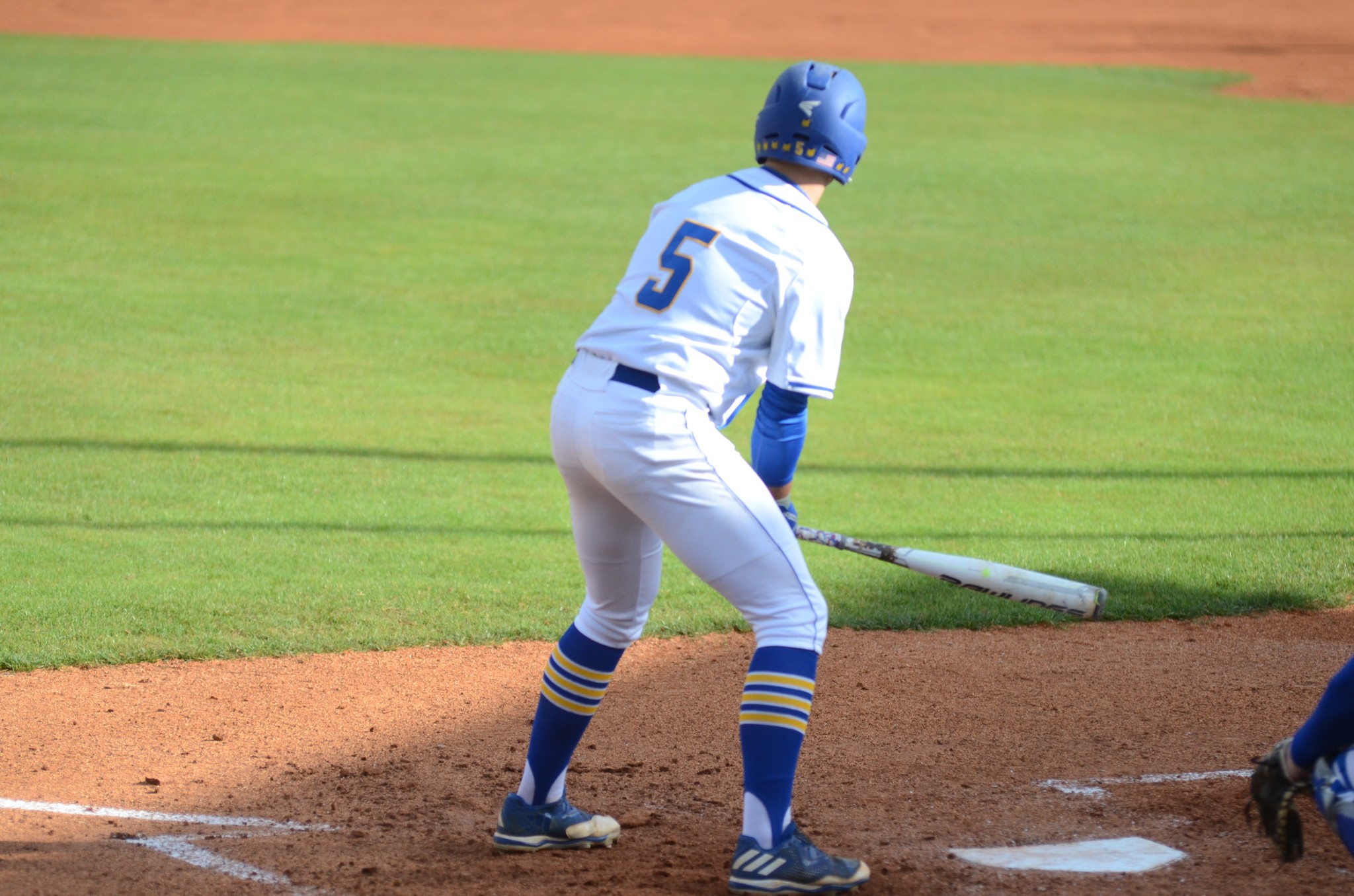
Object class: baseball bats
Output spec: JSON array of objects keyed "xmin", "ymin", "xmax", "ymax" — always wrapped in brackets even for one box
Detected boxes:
[{"xmin": 793, "ymin": 526, "xmax": 1108, "ymax": 626}]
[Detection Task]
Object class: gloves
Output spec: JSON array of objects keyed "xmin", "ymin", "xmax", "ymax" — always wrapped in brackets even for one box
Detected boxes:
[{"xmin": 779, "ymin": 499, "xmax": 801, "ymax": 537}]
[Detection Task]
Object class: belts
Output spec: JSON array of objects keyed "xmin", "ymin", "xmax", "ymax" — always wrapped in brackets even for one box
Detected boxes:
[{"xmin": 612, "ymin": 364, "xmax": 662, "ymax": 392}]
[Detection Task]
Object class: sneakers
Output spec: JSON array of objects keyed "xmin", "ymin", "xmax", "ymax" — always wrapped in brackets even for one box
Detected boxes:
[
  {"xmin": 490, "ymin": 791, "xmax": 621, "ymax": 853},
  {"xmin": 726, "ymin": 821, "xmax": 871, "ymax": 895}
]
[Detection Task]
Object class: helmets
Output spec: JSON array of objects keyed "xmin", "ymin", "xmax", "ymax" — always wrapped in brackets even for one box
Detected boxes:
[{"xmin": 753, "ymin": 63, "xmax": 867, "ymax": 186}]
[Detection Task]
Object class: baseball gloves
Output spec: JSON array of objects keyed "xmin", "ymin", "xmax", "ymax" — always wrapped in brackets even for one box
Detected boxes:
[{"xmin": 1249, "ymin": 737, "xmax": 1308, "ymax": 865}]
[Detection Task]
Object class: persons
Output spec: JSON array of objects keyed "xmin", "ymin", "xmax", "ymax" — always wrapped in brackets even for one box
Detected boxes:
[
  {"xmin": 489, "ymin": 62, "xmax": 867, "ymax": 896},
  {"xmin": 1242, "ymin": 656, "xmax": 1354, "ymax": 864}
]
